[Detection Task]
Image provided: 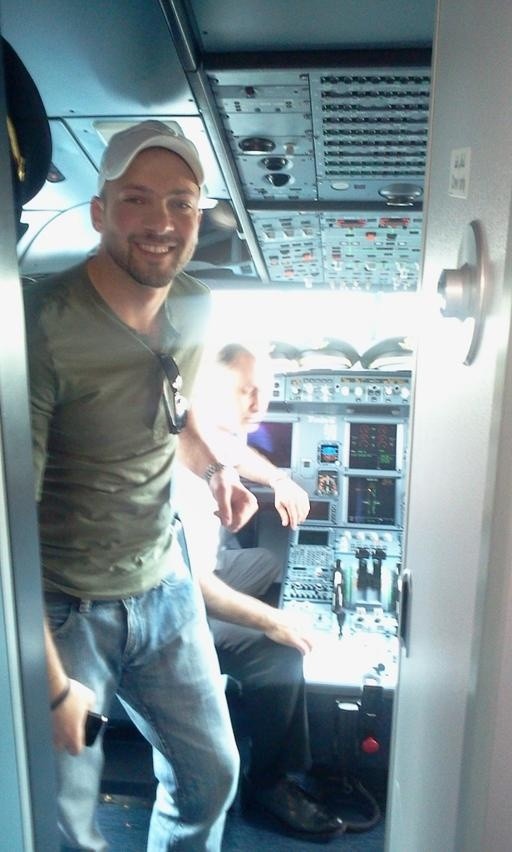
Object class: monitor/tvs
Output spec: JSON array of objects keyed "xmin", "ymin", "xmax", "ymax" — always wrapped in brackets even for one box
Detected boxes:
[{"xmin": 245, "ymin": 418, "xmax": 297, "ymax": 472}]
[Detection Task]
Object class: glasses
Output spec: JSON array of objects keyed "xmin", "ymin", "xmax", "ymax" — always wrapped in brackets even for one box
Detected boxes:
[{"xmin": 158, "ymin": 353, "xmax": 187, "ymax": 433}]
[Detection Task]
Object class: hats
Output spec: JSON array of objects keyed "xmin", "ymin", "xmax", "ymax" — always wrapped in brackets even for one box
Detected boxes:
[{"xmin": 97, "ymin": 120, "xmax": 204, "ymax": 196}]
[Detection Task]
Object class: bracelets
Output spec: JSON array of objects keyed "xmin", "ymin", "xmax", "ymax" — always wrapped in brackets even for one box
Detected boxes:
[
  {"xmin": 48, "ymin": 677, "xmax": 73, "ymax": 714},
  {"xmin": 202, "ymin": 459, "xmax": 241, "ymax": 487}
]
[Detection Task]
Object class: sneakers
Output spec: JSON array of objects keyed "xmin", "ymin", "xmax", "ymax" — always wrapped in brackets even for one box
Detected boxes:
[{"xmin": 264, "ymin": 782, "xmax": 347, "ymax": 840}]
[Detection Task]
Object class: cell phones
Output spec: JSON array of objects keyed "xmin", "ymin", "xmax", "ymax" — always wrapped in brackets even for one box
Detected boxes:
[{"xmin": 85, "ymin": 710, "xmax": 109, "ymax": 748}]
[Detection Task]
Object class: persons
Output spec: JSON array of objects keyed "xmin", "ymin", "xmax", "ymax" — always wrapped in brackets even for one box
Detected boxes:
[
  {"xmin": 21, "ymin": 121, "xmax": 317, "ymax": 851},
  {"xmin": 169, "ymin": 339, "xmax": 349, "ymax": 842}
]
